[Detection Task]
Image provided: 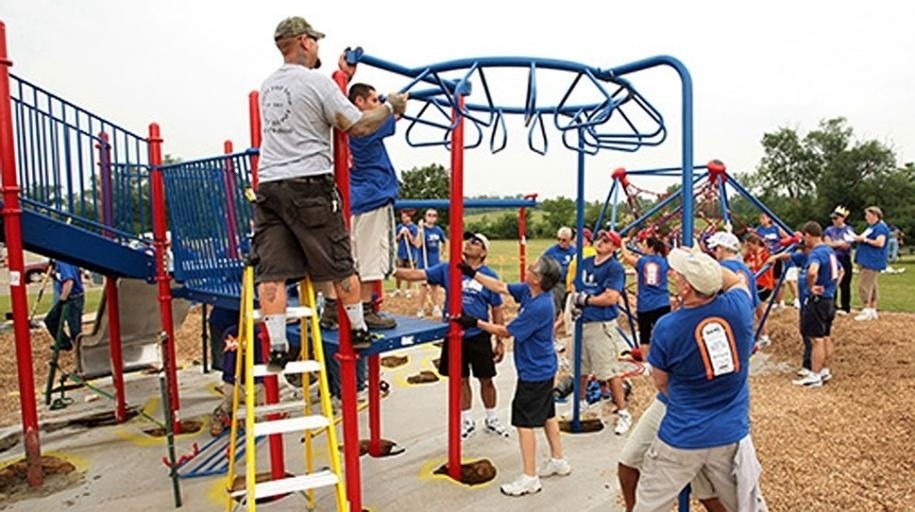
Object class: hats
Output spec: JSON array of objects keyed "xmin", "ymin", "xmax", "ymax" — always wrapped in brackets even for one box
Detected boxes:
[
  {"xmin": 463, "ymin": 230, "xmax": 490, "ymax": 251},
  {"xmin": 573, "ymin": 228, "xmax": 592, "ymax": 241},
  {"xmin": 598, "ymin": 230, "xmax": 622, "ymax": 247},
  {"xmin": 274, "ymin": 16, "xmax": 327, "ymax": 41},
  {"xmin": 707, "ymin": 231, "xmax": 741, "ymax": 252},
  {"xmin": 666, "ymin": 250, "xmax": 724, "ymax": 295}
]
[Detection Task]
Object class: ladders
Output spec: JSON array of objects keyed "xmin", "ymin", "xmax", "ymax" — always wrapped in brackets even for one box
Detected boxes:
[{"xmin": 223, "ymin": 260, "xmax": 349, "ymax": 512}]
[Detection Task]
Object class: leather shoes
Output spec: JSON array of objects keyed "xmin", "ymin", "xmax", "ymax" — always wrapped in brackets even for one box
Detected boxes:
[
  {"xmin": 267, "ymin": 350, "xmax": 288, "ymax": 373},
  {"xmin": 351, "ymin": 328, "xmax": 373, "ymax": 349}
]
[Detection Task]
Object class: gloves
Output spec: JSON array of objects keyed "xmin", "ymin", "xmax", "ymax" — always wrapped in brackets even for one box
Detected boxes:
[
  {"xmin": 456, "ymin": 258, "xmax": 477, "ymax": 280},
  {"xmin": 452, "ymin": 307, "xmax": 479, "ymax": 331}
]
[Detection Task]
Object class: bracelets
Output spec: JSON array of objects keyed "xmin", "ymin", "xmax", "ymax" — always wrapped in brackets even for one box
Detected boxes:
[{"xmin": 383, "ymin": 100, "xmax": 395, "ymax": 116}]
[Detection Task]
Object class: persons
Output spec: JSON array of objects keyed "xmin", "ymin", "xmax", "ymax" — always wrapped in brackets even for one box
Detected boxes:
[
  {"xmin": 44, "ymin": 255, "xmax": 85, "ymax": 352},
  {"xmin": 253, "ymin": 15, "xmax": 410, "ymax": 368},
  {"xmin": 212, "ymin": 204, "xmax": 905, "ymax": 512},
  {"xmin": 137, "ymin": 236, "xmax": 155, "ymax": 257},
  {"xmin": 316, "ymin": 80, "xmax": 405, "ymax": 331}
]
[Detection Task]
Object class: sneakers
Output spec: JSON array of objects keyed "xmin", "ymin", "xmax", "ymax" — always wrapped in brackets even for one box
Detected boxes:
[
  {"xmin": 793, "ymin": 368, "xmax": 831, "ymax": 387},
  {"xmin": 615, "ymin": 408, "xmax": 632, "ymax": 436},
  {"xmin": 461, "ymin": 418, "xmax": 477, "ymax": 441},
  {"xmin": 535, "ymin": 457, "xmax": 572, "ymax": 478},
  {"xmin": 318, "ymin": 304, "xmax": 341, "ymax": 331},
  {"xmin": 757, "ymin": 339, "xmax": 770, "ymax": 349},
  {"xmin": 362, "ymin": 306, "xmax": 398, "ymax": 330},
  {"xmin": 858, "ymin": 310, "xmax": 877, "ymax": 321},
  {"xmin": 485, "ymin": 419, "xmax": 509, "ymax": 438},
  {"xmin": 500, "ymin": 475, "xmax": 543, "ymax": 496}
]
